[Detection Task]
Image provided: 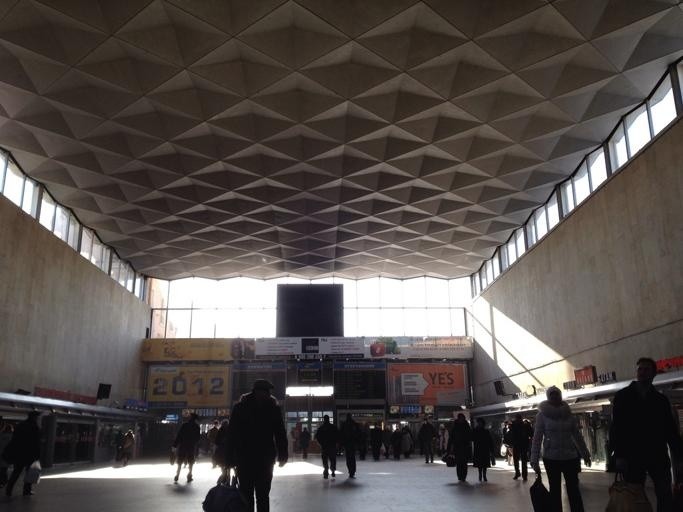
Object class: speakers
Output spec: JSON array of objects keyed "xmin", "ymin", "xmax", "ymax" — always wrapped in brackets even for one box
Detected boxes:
[
  {"xmin": 493, "ymin": 380, "xmax": 505, "ymax": 395},
  {"xmin": 97, "ymin": 382, "xmax": 112, "ymax": 399}
]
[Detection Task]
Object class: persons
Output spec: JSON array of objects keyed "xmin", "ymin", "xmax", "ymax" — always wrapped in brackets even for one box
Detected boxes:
[
  {"xmin": 116, "ymin": 429, "xmax": 133, "ymax": 463},
  {"xmin": 199, "ymin": 423, "xmax": 219, "ymax": 456},
  {"xmin": 222, "ymin": 378, "xmax": 288, "ymax": 512},
  {"xmin": 316, "ymin": 412, "xmax": 360, "ymax": 478},
  {"xmin": 529, "ymin": 384, "xmax": 591, "ymax": 511},
  {"xmin": 175, "ymin": 412, "xmax": 200, "ymax": 483},
  {"xmin": 611, "ymin": 356, "xmax": 683, "ymax": 511},
  {"xmin": 360, "ymin": 417, "xmax": 437, "ymax": 463},
  {"xmin": 302, "ymin": 429, "xmax": 310, "ymax": 459},
  {"xmin": 0, "ymin": 410, "xmax": 53, "ymax": 497},
  {"xmin": 446, "ymin": 412, "xmax": 535, "ymax": 483}
]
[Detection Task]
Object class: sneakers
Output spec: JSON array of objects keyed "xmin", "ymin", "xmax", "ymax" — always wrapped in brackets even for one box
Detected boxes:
[
  {"xmin": 173, "ymin": 475, "xmax": 194, "ymax": 482},
  {"xmin": 457, "ymin": 473, "xmax": 528, "ymax": 482},
  {"xmin": 324, "ymin": 472, "xmax": 357, "ymax": 479},
  {"xmin": 22, "ymin": 490, "xmax": 36, "ymax": 496}
]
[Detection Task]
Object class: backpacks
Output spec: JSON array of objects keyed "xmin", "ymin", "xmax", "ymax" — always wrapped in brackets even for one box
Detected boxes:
[
  {"xmin": 252, "ymin": 378, "xmax": 276, "ymax": 390},
  {"xmin": 530, "ymin": 469, "xmax": 556, "ymax": 512}
]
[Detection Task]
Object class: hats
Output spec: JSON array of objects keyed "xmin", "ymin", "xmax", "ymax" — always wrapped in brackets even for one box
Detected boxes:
[
  {"xmin": 635, "ymin": 356, "xmax": 657, "ymax": 365},
  {"xmin": 545, "ymin": 386, "xmax": 562, "ymax": 397}
]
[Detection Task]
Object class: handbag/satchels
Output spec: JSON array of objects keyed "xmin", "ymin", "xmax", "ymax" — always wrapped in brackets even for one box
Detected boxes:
[
  {"xmin": 604, "ymin": 470, "xmax": 653, "ymax": 512},
  {"xmin": 446, "ymin": 454, "xmax": 456, "ymax": 467},
  {"xmin": 202, "ymin": 464, "xmax": 253, "ymax": 512}
]
